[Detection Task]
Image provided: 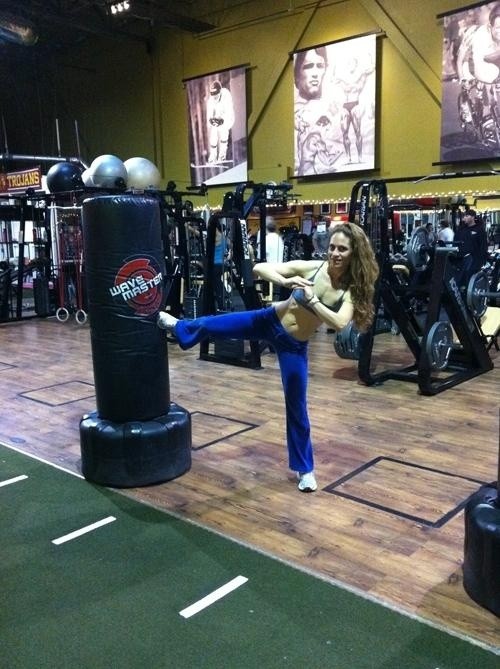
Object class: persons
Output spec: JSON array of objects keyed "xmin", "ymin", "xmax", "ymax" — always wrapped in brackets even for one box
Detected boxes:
[
  {"xmin": 204, "ymin": 79, "xmax": 236, "ymax": 166},
  {"xmin": 333, "ymin": 56, "xmax": 376, "ymax": 165},
  {"xmin": 295, "ymin": 45, "xmax": 329, "ymax": 101},
  {"xmin": 296, "ymin": 114, "xmax": 342, "ymax": 176},
  {"xmin": 443, "ymin": 4, "xmax": 500, "ymax": 102},
  {"xmin": 156, "ymin": 222, "xmax": 382, "ymax": 495}
]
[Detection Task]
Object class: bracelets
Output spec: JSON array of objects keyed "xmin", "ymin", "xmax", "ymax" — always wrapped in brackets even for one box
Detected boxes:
[{"xmin": 307, "ymin": 300, "xmax": 321, "ymax": 308}]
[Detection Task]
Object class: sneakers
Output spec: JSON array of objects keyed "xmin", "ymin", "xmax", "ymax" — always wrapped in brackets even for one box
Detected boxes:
[
  {"xmin": 296, "ymin": 470, "xmax": 318, "ymax": 492},
  {"xmin": 156, "ymin": 310, "xmax": 180, "ymax": 331}
]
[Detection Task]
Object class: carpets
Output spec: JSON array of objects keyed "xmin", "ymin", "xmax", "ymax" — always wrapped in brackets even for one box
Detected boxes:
[{"xmin": 0, "ymin": 437, "xmax": 500, "ymax": 668}]
